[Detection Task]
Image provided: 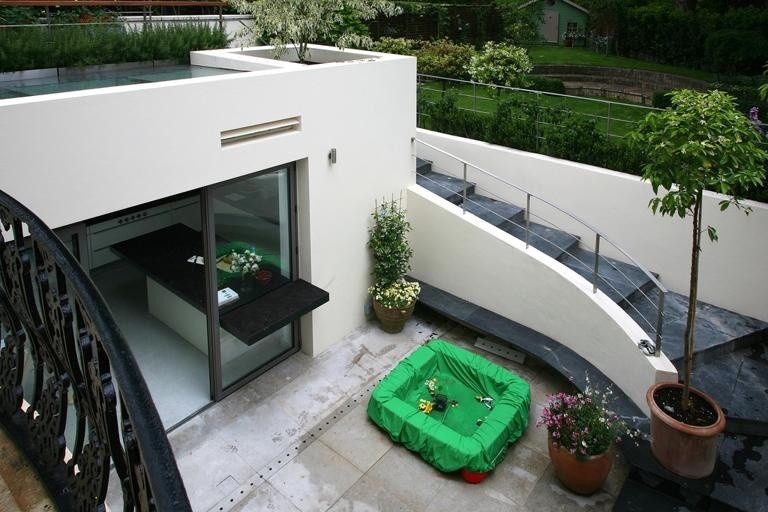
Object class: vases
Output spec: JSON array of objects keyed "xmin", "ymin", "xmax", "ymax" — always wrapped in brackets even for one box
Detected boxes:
[
  {"xmin": 373, "ymin": 296, "xmax": 416, "ymax": 334},
  {"xmin": 547, "ymin": 429, "xmax": 616, "ymax": 494}
]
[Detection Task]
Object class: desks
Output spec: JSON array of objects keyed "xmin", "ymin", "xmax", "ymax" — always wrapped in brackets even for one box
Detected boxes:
[{"xmin": 108, "ymin": 221, "xmax": 329, "ymax": 367}]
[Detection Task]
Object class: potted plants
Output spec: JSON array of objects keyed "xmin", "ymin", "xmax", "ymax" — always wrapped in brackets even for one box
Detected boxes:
[{"xmin": 623, "ymin": 86, "xmax": 768, "ymax": 480}]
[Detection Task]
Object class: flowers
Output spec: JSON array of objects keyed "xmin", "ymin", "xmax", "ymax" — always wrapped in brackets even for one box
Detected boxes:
[
  {"xmin": 535, "ymin": 370, "xmax": 648, "ymax": 464},
  {"xmin": 367, "ymin": 191, "xmax": 421, "ymax": 313}
]
